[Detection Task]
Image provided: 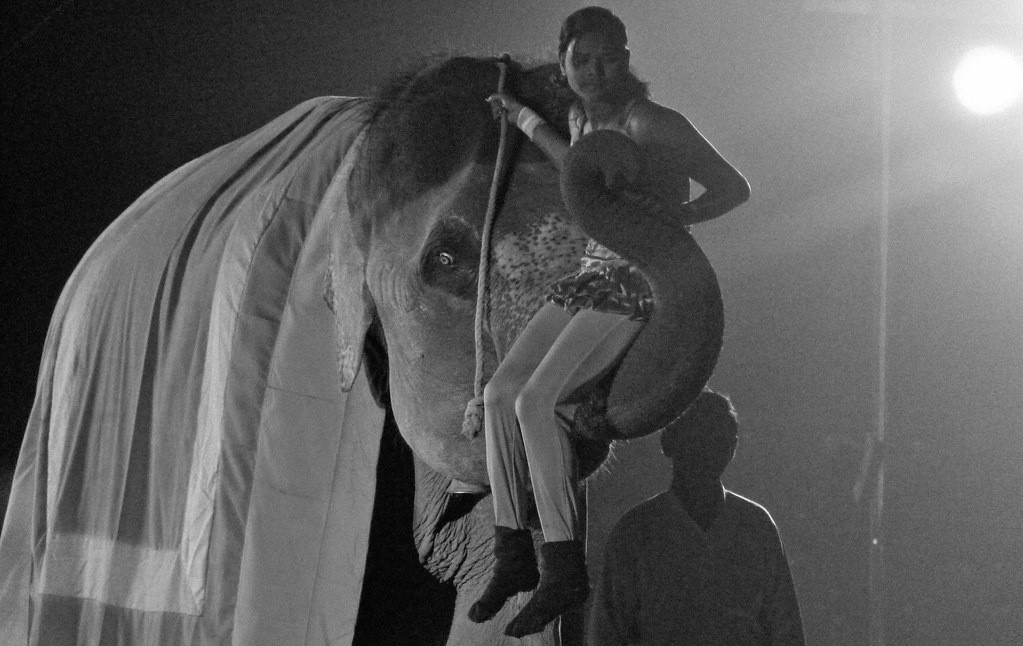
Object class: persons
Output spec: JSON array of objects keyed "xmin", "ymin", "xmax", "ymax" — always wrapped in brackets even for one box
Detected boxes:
[
  {"xmin": 467, "ymin": 7, "xmax": 751, "ymax": 638},
  {"xmin": 587, "ymin": 393, "xmax": 805, "ymax": 646}
]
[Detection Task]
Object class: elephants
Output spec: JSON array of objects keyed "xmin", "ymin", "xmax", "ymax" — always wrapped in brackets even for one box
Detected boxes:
[{"xmin": 0, "ymin": 53, "xmax": 722, "ymax": 646}]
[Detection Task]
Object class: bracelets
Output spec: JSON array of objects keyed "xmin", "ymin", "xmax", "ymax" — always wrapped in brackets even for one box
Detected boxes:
[{"xmin": 518, "ymin": 107, "xmax": 545, "ymax": 142}]
